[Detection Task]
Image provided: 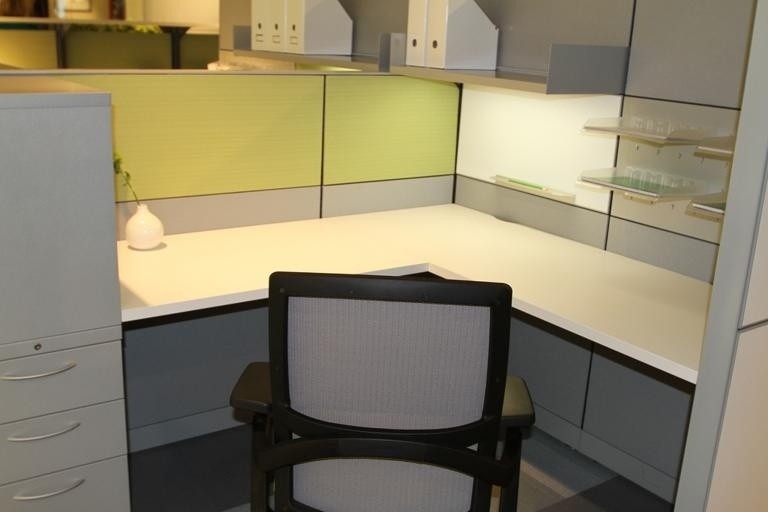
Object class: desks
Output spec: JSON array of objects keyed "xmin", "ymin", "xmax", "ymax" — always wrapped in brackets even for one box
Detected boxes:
[
  {"xmin": 430, "ymin": 222, "xmax": 714, "ymax": 395},
  {"xmin": 116, "ymin": 200, "xmax": 509, "ymax": 325}
]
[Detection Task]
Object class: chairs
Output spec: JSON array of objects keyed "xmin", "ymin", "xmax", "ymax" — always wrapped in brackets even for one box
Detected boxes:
[{"xmin": 230, "ymin": 272, "xmax": 536, "ymax": 511}]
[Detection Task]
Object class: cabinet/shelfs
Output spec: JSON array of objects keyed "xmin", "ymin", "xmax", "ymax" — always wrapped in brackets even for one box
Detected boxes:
[
  {"xmin": 491, "ymin": 120, "xmax": 734, "ymax": 232},
  {"xmin": 1, "ymin": 92, "xmax": 133, "ymax": 511}
]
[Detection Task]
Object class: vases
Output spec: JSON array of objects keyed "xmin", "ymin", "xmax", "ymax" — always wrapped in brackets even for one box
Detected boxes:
[{"xmin": 127, "ymin": 202, "xmax": 165, "ymax": 253}]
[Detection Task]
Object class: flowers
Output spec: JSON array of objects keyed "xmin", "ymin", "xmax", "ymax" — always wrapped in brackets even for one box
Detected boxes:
[{"xmin": 115, "ymin": 156, "xmax": 142, "ymax": 204}]
